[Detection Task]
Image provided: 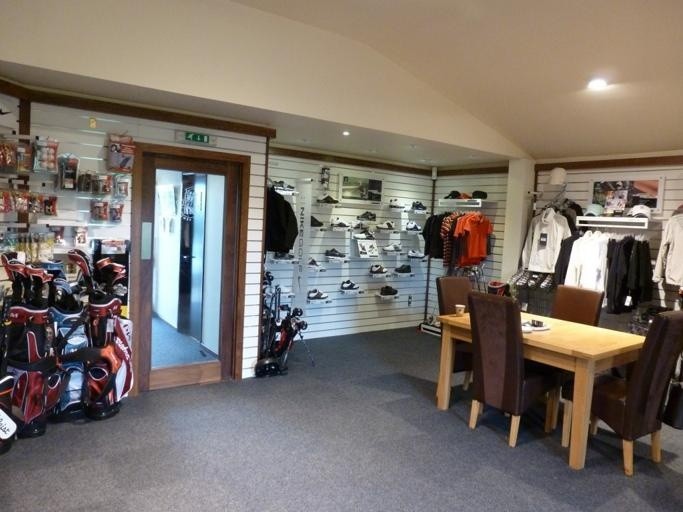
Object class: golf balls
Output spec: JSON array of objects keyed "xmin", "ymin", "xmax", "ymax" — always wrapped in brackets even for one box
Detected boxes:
[{"xmin": 39, "ymin": 147, "xmax": 55, "ymax": 171}]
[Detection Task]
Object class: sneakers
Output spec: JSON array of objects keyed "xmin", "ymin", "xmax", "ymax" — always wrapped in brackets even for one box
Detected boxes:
[
  {"xmin": 273, "ymin": 181, "xmax": 294, "ymax": 191},
  {"xmin": 311, "ymin": 196, "xmax": 426, "ymax": 239},
  {"xmin": 275, "ymin": 250, "xmax": 294, "ymax": 259},
  {"xmin": 307, "ymin": 242, "xmax": 425, "ymax": 299}
]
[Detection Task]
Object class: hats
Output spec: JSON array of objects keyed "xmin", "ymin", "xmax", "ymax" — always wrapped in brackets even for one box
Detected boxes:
[
  {"xmin": 584, "ymin": 204, "xmax": 604, "ymax": 216},
  {"xmin": 632, "ymin": 205, "xmax": 651, "ymax": 218},
  {"xmin": 549, "ymin": 167, "xmax": 567, "ymax": 185}
]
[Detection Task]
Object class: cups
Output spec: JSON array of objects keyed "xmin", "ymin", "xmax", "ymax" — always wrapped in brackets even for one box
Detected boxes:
[{"xmin": 454, "ymin": 304, "xmax": 466, "ymax": 317}]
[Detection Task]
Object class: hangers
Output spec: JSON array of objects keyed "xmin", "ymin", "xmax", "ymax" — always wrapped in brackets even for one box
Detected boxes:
[
  {"xmin": 579, "ymin": 222, "xmax": 646, "ymax": 237},
  {"xmin": 543, "ymin": 187, "xmax": 567, "ymax": 211},
  {"xmin": 440, "ymin": 204, "xmax": 485, "ymax": 212}
]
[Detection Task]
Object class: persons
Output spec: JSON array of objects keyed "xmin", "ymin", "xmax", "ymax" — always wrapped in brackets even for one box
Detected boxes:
[
  {"xmin": 613, "ymin": 182, "xmax": 624, "ymax": 189},
  {"xmin": 357, "ymin": 181, "xmax": 366, "ymax": 197}
]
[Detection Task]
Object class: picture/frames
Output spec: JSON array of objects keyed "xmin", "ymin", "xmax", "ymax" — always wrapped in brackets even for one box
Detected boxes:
[
  {"xmin": 338, "ymin": 175, "xmax": 385, "ymax": 207},
  {"xmin": 589, "ymin": 177, "xmax": 666, "ymax": 214}
]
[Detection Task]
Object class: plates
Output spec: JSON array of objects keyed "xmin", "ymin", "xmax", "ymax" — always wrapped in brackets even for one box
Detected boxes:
[{"xmin": 521, "ymin": 320, "xmax": 551, "ymax": 333}]
